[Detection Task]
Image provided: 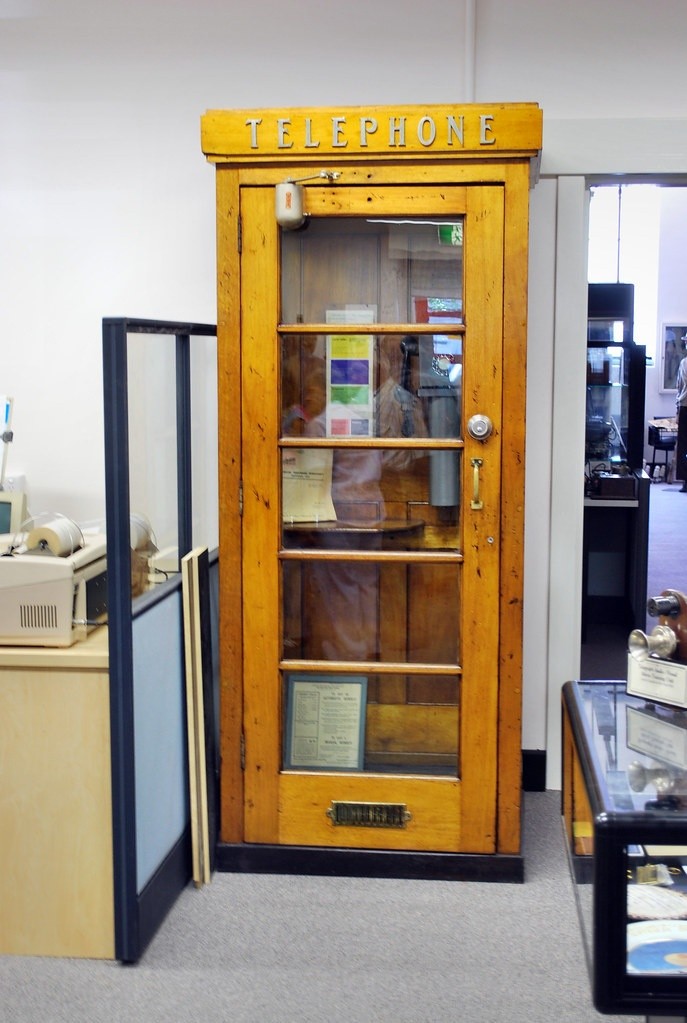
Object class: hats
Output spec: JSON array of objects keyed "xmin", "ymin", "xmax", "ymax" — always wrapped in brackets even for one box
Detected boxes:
[{"xmin": 681, "ymin": 333, "xmax": 687, "ymax": 340}]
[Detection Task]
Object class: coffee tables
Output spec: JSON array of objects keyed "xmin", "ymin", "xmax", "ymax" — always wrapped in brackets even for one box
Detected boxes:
[{"xmin": 561, "ymin": 679, "xmax": 687, "ymax": 1017}]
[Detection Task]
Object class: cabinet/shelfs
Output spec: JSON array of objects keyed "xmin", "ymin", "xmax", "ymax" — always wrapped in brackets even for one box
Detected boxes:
[{"xmin": 579, "ymin": 282, "xmax": 650, "ymax": 680}]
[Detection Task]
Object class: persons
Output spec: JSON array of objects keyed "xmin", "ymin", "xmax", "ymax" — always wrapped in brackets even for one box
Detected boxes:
[
  {"xmin": 676, "ymin": 334, "xmax": 687, "ymax": 492},
  {"xmin": 280, "ymin": 333, "xmax": 460, "ymax": 685}
]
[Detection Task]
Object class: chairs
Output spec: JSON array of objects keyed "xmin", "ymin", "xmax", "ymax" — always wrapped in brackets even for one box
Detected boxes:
[{"xmin": 650, "ymin": 416, "xmax": 675, "ymax": 482}]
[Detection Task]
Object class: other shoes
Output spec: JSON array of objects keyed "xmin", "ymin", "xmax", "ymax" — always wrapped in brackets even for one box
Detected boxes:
[{"xmin": 679, "ymin": 484, "xmax": 687, "ymax": 492}]
